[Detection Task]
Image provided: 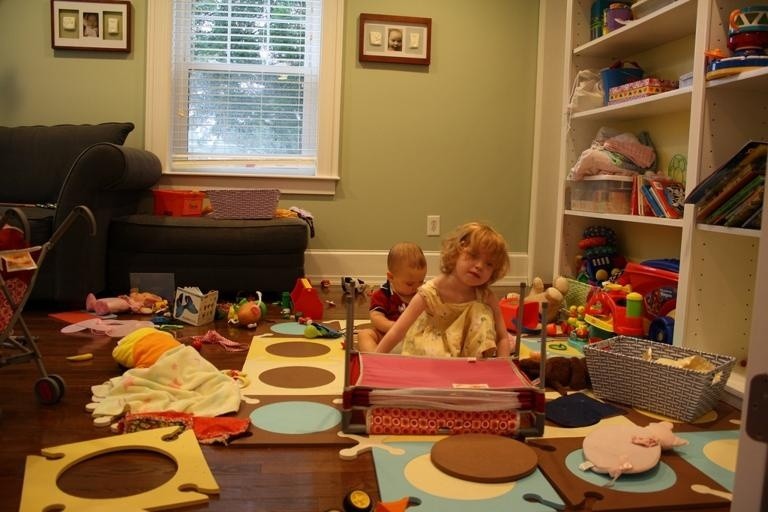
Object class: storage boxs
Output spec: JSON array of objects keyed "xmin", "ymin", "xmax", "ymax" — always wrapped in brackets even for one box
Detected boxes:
[
  {"xmin": 152, "ymin": 189, "xmax": 205, "ymax": 216},
  {"xmin": 566, "ymin": 175, "xmax": 634, "ymax": 214}
]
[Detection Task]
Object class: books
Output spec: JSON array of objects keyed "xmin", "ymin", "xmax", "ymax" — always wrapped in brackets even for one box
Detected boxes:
[{"xmin": 630, "ymin": 139, "xmax": 768, "ymax": 230}]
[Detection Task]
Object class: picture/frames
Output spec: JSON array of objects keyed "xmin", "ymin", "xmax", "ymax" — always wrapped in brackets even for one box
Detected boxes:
[
  {"xmin": 50, "ymin": 0, "xmax": 132, "ymax": 53},
  {"xmin": 359, "ymin": 13, "xmax": 432, "ymax": 65}
]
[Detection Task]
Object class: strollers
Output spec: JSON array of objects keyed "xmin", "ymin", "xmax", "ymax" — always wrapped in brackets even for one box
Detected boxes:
[{"xmin": 0, "ymin": 203, "xmax": 97, "ymax": 405}]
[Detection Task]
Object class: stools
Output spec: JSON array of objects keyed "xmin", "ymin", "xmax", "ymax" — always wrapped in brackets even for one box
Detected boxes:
[{"xmin": 109, "ymin": 212, "xmax": 308, "ymax": 303}]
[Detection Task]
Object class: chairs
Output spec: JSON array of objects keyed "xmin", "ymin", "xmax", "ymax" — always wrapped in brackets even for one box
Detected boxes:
[{"xmin": 0, "ymin": 122, "xmax": 162, "ymax": 301}]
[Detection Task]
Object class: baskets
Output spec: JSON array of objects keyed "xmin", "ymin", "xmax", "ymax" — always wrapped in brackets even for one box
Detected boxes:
[{"xmin": 584, "ymin": 335, "xmax": 737, "ymax": 422}]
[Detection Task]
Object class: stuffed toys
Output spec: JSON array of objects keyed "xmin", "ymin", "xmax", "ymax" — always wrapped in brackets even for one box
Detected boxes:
[
  {"xmin": 85, "ymin": 276, "xmax": 375, "ymax": 351},
  {"xmin": 521, "ymin": 357, "xmax": 591, "ymax": 396},
  {"xmin": 113, "ymin": 327, "xmax": 184, "ymax": 370},
  {"xmin": 499, "ymin": 224, "xmax": 680, "ymax": 352}
]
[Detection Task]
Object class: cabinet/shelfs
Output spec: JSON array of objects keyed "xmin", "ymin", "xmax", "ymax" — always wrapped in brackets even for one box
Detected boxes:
[{"xmin": 552, "ymin": 0, "xmax": 768, "ymax": 411}]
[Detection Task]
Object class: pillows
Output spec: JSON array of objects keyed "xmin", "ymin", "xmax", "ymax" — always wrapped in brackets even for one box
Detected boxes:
[{"xmin": 0, "ymin": 122, "xmax": 135, "ymax": 202}]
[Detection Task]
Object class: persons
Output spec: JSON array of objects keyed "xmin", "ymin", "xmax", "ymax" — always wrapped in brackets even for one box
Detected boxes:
[
  {"xmin": 388, "ymin": 30, "xmax": 402, "ymax": 52},
  {"xmin": 84, "ymin": 14, "xmax": 99, "ymax": 37},
  {"xmin": 358, "ymin": 243, "xmax": 429, "ymax": 353},
  {"xmin": 378, "ymin": 222, "xmax": 512, "ymax": 358}
]
